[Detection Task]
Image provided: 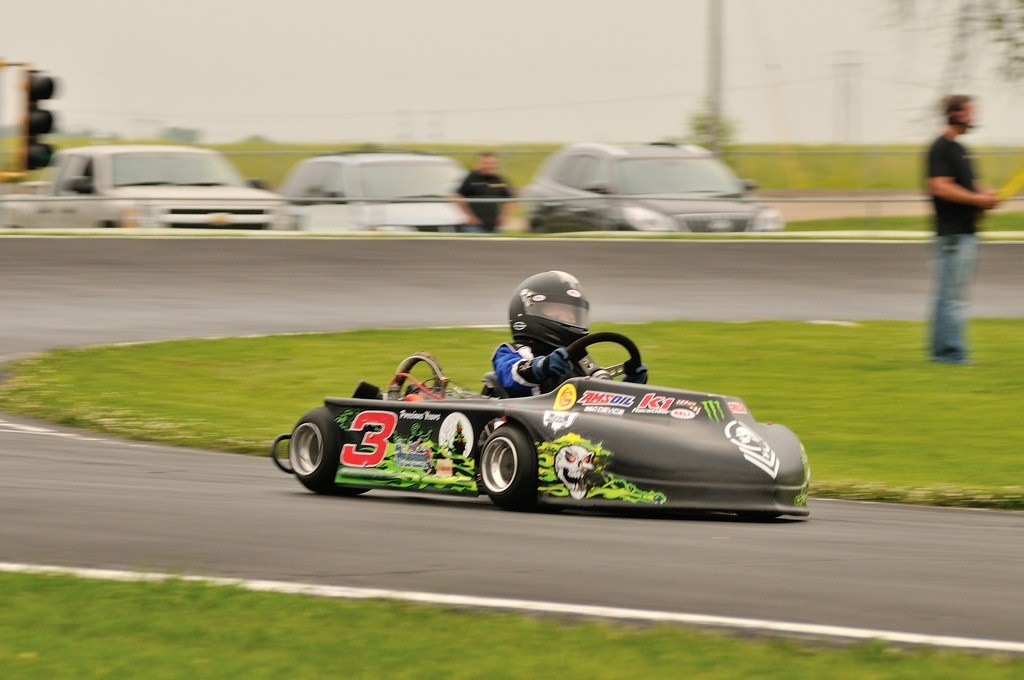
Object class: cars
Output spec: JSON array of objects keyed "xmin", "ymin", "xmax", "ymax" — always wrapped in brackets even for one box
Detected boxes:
[{"xmin": 276, "ymin": 153, "xmax": 478, "ymax": 231}]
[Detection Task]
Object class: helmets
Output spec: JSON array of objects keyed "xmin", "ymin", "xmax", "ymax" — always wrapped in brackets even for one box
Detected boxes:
[{"xmin": 508, "ymin": 271, "xmax": 589, "ymax": 351}]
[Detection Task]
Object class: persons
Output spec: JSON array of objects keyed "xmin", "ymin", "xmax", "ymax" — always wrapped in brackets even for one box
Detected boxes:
[
  {"xmin": 492, "ymin": 271, "xmax": 648, "ymax": 398},
  {"xmin": 450, "ymin": 155, "xmax": 518, "ymax": 233},
  {"xmin": 924, "ymin": 94, "xmax": 1002, "ymax": 362}
]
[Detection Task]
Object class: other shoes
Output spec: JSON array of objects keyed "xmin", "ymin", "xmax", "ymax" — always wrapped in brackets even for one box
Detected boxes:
[{"xmin": 931, "ymin": 347, "xmax": 968, "ymax": 363}]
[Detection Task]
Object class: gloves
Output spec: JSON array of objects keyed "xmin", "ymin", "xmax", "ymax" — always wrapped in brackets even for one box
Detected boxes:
[
  {"xmin": 623, "ymin": 365, "xmax": 648, "ymax": 384},
  {"xmin": 532, "ymin": 347, "xmax": 574, "ymax": 383}
]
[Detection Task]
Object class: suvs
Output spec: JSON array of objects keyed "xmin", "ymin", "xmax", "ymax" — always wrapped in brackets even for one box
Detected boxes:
[{"xmin": 521, "ymin": 142, "xmax": 784, "ymax": 231}]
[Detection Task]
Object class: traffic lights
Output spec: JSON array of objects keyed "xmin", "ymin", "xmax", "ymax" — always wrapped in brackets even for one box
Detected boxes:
[{"xmin": 20, "ymin": 68, "xmax": 56, "ymax": 172}]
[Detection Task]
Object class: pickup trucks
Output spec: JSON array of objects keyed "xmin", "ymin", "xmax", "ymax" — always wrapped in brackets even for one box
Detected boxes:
[{"xmin": 0, "ymin": 142, "xmax": 294, "ymax": 232}]
